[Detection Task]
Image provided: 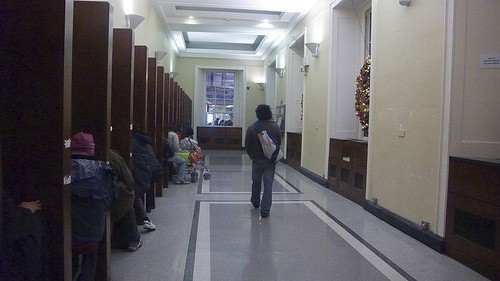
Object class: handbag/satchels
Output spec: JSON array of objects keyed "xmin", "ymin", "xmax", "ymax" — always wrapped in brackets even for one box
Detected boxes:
[{"xmin": 253, "ymin": 121, "xmax": 284, "ymax": 166}]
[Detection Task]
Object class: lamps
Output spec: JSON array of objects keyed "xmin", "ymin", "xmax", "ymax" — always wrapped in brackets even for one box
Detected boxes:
[
  {"xmin": 305, "ymin": 42, "xmax": 320, "ymax": 58},
  {"xmin": 274, "ymin": 68, "xmax": 283, "ymax": 78},
  {"xmin": 257, "ymin": 83, "xmax": 264, "ymax": 90},
  {"xmin": 128, "ymin": 14, "xmax": 145, "ymax": 30},
  {"xmin": 155, "ymin": 51, "xmax": 167, "ymax": 64}
]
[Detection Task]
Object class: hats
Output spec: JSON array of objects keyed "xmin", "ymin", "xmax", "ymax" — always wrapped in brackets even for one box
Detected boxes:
[
  {"xmin": 254, "ymin": 104, "xmax": 273, "ymax": 121},
  {"xmin": 71, "ymin": 131, "xmax": 94, "ymax": 157}
]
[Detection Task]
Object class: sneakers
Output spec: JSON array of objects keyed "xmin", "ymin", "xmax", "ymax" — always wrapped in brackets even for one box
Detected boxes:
[{"xmin": 143, "ymin": 220, "xmax": 156, "ymax": 230}]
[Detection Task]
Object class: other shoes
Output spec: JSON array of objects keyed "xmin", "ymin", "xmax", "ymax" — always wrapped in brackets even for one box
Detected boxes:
[
  {"xmin": 260, "ymin": 213, "xmax": 269, "ymax": 218},
  {"xmin": 251, "ymin": 202, "xmax": 260, "ymax": 208},
  {"xmin": 175, "ymin": 178, "xmax": 190, "ymax": 185},
  {"xmin": 128, "ymin": 237, "xmax": 143, "ymax": 252}
]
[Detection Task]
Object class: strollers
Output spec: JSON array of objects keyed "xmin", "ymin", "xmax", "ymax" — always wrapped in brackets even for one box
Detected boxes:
[{"xmin": 184, "ymin": 136, "xmax": 211, "ymax": 183}]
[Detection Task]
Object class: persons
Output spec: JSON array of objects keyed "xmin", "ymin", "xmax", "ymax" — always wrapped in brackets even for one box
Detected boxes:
[
  {"xmin": 162, "ymin": 122, "xmax": 204, "ymax": 184},
  {"xmin": 132, "ymin": 121, "xmax": 161, "ymax": 230},
  {"xmin": 214, "ymin": 118, "xmax": 233, "ymax": 126},
  {"xmin": 245, "ymin": 104, "xmax": 281, "ymax": 218},
  {"xmin": 0, "ymin": 187, "xmax": 44, "ymax": 281},
  {"xmin": 110, "ymin": 149, "xmax": 143, "ymax": 252},
  {"xmin": 70, "ymin": 132, "xmax": 116, "ymax": 281}
]
